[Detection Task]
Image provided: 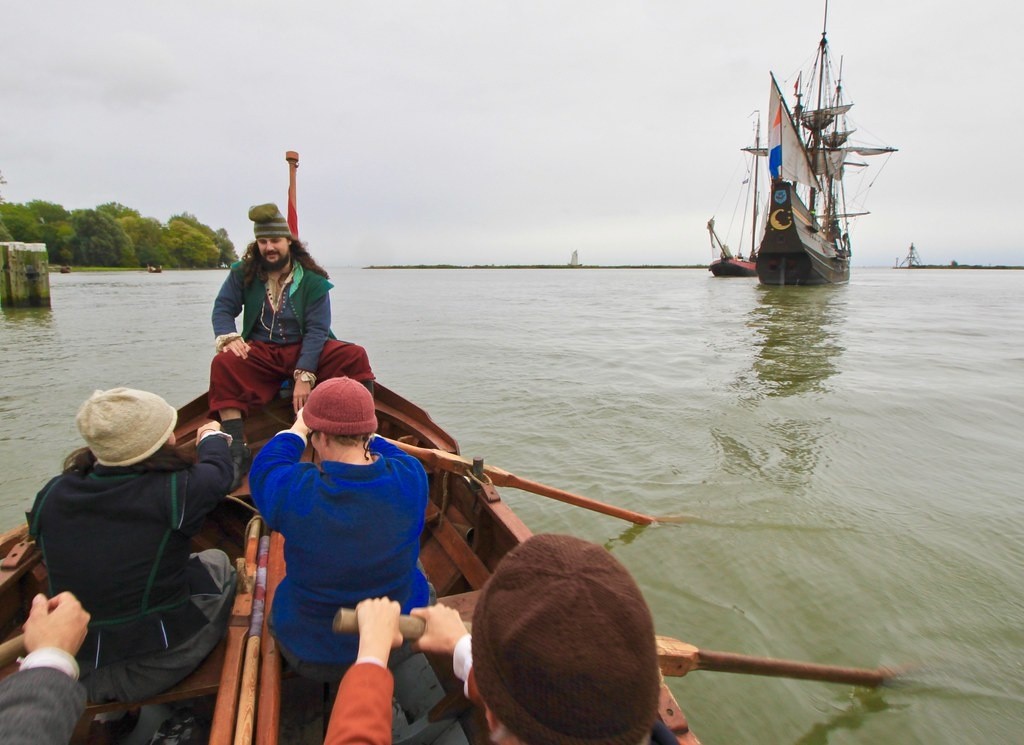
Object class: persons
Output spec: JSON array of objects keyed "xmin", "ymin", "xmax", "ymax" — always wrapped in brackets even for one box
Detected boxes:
[
  {"xmin": 324, "ymin": 532, "xmax": 678, "ymax": 745},
  {"xmin": 249, "ymin": 376, "xmax": 437, "ymax": 682},
  {"xmin": 206, "ymin": 205, "xmax": 375, "ymax": 493},
  {"xmin": 0, "ymin": 590, "xmax": 90, "ymax": 745},
  {"xmin": 27, "ymin": 387, "xmax": 236, "ymax": 701}
]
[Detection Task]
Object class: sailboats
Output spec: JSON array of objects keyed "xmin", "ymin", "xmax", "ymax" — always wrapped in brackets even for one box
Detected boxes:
[
  {"xmin": 706, "ymin": 111, "xmax": 770, "ymax": 278},
  {"xmin": 743, "ymin": 0, "xmax": 899, "ymax": 286}
]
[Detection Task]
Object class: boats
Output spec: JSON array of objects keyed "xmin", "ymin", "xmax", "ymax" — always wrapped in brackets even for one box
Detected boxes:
[
  {"xmin": 147, "ymin": 265, "xmax": 162, "ymax": 273},
  {"xmin": 59, "ymin": 266, "xmax": 71, "ymax": 273}
]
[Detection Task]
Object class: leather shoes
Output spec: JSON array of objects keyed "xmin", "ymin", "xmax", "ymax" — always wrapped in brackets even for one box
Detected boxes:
[{"xmin": 230, "ymin": 445, "xmax": 254, "ymax": 494}]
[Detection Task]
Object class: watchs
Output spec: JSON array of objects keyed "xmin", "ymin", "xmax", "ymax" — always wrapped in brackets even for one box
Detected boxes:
[{"xmin": 301, "ymin": 374, "xmax": 311, "ymax": 382}]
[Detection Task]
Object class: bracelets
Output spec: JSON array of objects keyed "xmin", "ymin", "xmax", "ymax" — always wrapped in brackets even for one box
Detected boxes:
[{"xmin": 200, "ymin": 429, "xmax": 215, "ymax": 438}]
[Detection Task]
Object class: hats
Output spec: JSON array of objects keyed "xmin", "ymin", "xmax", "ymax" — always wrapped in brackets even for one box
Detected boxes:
[
  {"xmin": 249, "ymin": 202, "xmax": 293, "ymax": 242},
  {"xmin": 303, "ymin": 376, "xmax": 379, "ymax": 436},
  {"xmin": 471, "ymin": 532, "xmax": 663, "ymax": 745},
  {"xmin": 75, "ymin": 386, "xmax": 178, "ymax": 467}
]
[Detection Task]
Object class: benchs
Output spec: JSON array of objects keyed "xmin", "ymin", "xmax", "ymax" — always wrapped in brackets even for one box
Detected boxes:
[{"xmin": 0, "ymin": 589, "xmax": 483, "ymax": 713}]
